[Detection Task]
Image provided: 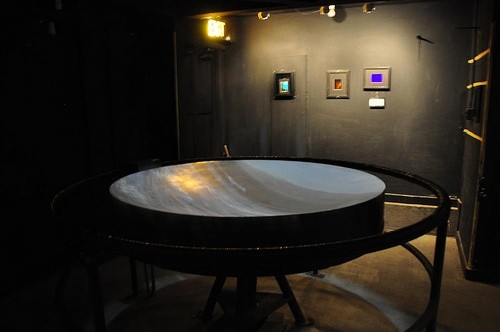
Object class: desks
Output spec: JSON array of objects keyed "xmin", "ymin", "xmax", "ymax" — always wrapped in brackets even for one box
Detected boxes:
[{"xmin": 51, "ymin": 155, "xmax": 461, "ymax": 332}]
